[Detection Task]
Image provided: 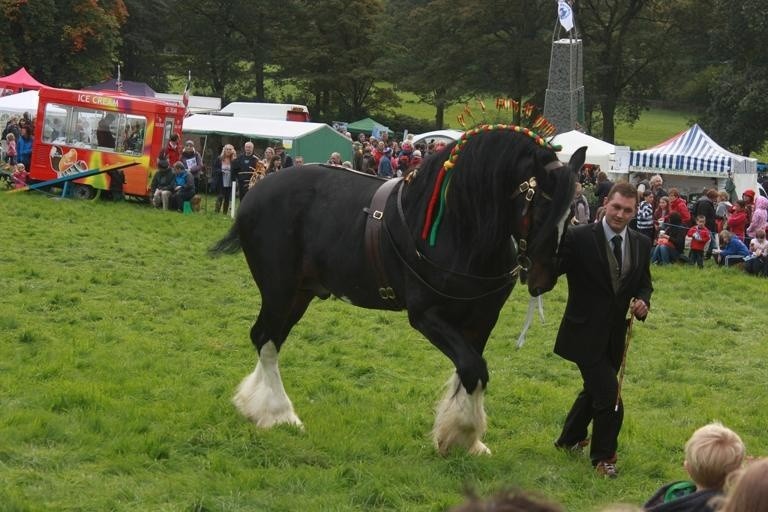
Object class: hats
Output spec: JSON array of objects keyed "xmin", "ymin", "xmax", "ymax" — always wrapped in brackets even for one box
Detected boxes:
[
  {"xmin": 743, "ymin": 190, "xmax": 755, "ymax": 201},
  {"xmin": 383, "ymin": 147, "xmax": 393, "ymax": 156}
]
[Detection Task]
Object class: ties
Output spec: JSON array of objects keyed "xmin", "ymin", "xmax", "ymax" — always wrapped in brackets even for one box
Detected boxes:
[{"xmin": 610, "ymin": 235, "xmax": 624, "ymax": 278}]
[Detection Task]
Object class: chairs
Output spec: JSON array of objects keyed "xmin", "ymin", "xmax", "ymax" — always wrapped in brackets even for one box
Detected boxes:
[{"xmin": 183, "ymin": 176, "xmax": 200, "ymax": 214}]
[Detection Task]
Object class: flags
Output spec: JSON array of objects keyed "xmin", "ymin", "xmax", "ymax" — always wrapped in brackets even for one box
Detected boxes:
[
  {"xmin": 558, "ymin": 1, "xmax": 573, "ymax": 31},
  {"xmin": 182, "ymin": 78, "xmax": 191, "ymax": 106}
]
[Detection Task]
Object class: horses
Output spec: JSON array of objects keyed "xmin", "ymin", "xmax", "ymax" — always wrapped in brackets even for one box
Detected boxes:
[{"xmin": 207, "ymin": 122, "xmax": 588, "ymax": 460}]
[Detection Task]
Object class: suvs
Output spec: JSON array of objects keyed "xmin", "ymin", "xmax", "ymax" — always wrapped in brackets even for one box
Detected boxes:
[{"xmin": 221, "ymin": 102, "xmax": 311, "ymax": 122}]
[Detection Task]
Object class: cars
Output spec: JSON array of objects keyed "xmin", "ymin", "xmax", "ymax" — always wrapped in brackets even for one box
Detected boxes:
[{"xmin": 412, "ymin": 130, "xmax": 465, "ymax": 147}]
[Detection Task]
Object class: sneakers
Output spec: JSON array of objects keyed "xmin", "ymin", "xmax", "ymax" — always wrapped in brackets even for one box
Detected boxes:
[
  {"xmin": 554, "ymin": 436, "xmax": 591, "ymax": 459},
  {"xmin": 595, "ymin": 454, "xmax": 621, "ymax": 482}
]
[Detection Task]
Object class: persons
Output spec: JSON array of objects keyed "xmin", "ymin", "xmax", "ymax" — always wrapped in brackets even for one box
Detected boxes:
[
  {"xmin": 142, "ymin": 134, "xmax": 304, "ymax": 217},
  {"xmin": 556, "ymin": 182, "xmax": 655, "ymax": 478},
  {"xmin": 633, "ymin": 171, "xmax": 767, "ymax": 275},
  {"xmin": 1, "ymin": 112, "xmax": 93, "ymax": 190},
  {"xmin": 98, "ymin": 114, "xmax": 115, "ymax": 148},
  {"xmin": 709, "ymin": 454, "xmax": 767, "ymax": 512},
  {"xmin": 643, "ymin": 421, "xmax": 744, "ymax": 512},
  {"xmin": 446, "ymin": 481, "xmax": 561, "ymax": 512},
  {"xmin": 328, "ymin": 131, "xmax": 446, "ymax": 182},
  {"xmin": 592, "ymin": 171, "xmax": 613, "ymax": 204}
]
